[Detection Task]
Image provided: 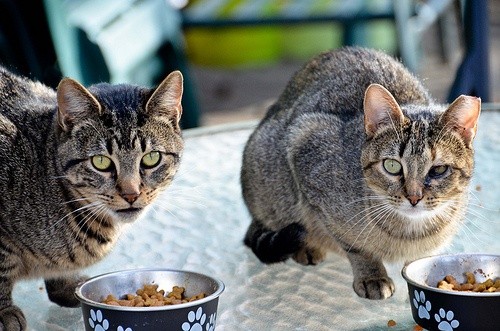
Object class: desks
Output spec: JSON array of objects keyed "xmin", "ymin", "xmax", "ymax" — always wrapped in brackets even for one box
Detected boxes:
[{"xmin": 10, "ymin": 100, "xmax": 500, "ymax": 331}]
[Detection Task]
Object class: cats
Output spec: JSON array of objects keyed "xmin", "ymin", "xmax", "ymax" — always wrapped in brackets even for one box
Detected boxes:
[
  {"xmin": 240, "ymin": 46, "xmax": 482, "ymax": 301},
  {"xmin": 0, "ymin": 64, "xmax": 186, "ymax": 331}
]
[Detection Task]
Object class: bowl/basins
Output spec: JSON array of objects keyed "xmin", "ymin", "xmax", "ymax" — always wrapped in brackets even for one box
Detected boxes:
[
  {"xmin": 73, "ymin": 267, "xmax": 225, "ymax": 331},
  {"xmin": 401, "ymin": 252, "xmax": 500, "ymax": 331}
]
